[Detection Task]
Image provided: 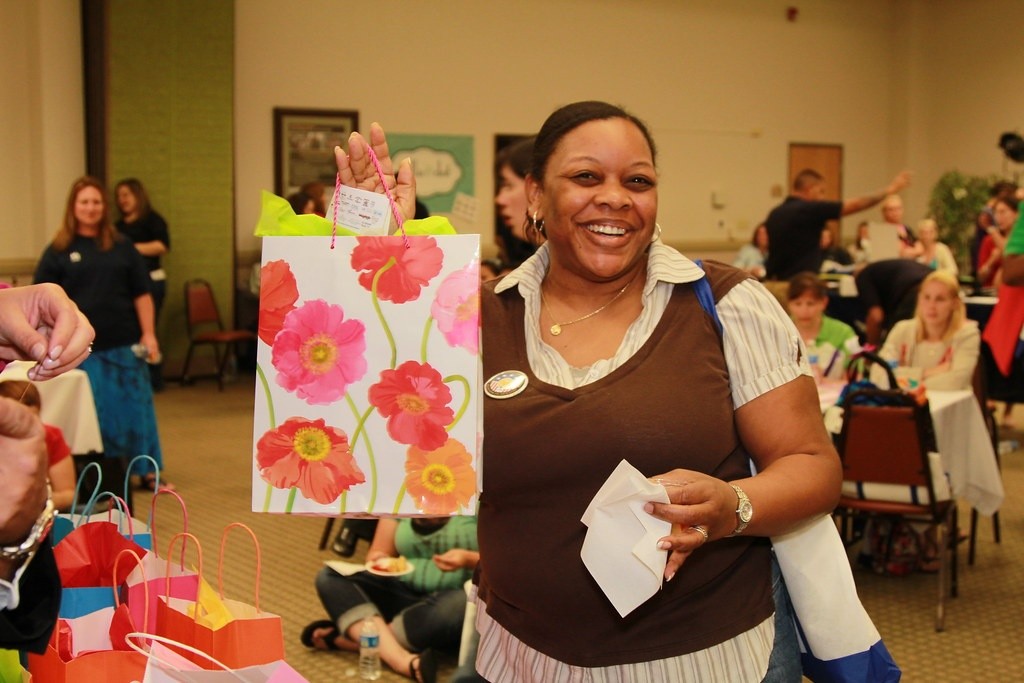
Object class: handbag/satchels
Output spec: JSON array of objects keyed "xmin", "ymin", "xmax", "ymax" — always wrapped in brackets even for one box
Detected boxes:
[
  {"xmin": 693, "ymin": 260, "xmax": 900, "ymax": 683},
  {"xmin": 0, "ymin": 454, "xmax": 308, "ymax": 682},
  {"xmin": 834, "ymin": 347, "xmax": 927, "ymax": 460},
  {"xmin": 250, "ymin": 137, "xmax": 484, "ymax": 518}
]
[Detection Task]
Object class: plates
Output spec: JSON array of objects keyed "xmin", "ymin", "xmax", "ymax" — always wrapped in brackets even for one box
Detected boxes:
[{"xmin": 365, "ymin": 558, "xmax": 414, "ymax": 577}]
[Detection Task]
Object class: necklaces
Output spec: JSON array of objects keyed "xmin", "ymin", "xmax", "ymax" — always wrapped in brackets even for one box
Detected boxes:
[{"xmin": 541, "ymin": 270, "xmax": 631, "ymax": 336}]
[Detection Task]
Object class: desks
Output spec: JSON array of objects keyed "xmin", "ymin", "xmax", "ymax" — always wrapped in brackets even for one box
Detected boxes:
[{"xmin": 822, "ymin": 295, "xmax": 1024, "ymax": 406}]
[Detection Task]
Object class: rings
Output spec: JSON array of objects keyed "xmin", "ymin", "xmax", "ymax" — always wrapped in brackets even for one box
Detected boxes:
[
  {"xmin": 89, "ymin": 341, "xmax": 93, "ymax": 355},
  {"xmin": 689, "ymin": 527, "xmax": 707, "ymax": 547}
]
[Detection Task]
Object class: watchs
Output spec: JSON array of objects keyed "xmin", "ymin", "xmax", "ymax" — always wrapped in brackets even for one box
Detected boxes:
[{"xmin": 1, "ymin": 475, "xmax": 54, "ymax": 560}]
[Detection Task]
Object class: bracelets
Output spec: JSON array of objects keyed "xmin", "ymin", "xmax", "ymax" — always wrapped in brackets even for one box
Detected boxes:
[{"xmin": 725, "ymin": 482, "xmax": 752, "ymax": 539}]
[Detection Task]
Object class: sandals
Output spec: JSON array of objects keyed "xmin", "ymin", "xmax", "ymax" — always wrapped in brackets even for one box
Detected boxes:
[{"xmin": 915, "ymin": 522, "xmax": 967, "ymax": 573}]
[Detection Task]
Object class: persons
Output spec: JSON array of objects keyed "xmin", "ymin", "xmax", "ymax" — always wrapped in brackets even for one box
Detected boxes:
[
  {"xmin": 301, "ymin": 474, "xmax": 493, "ymax": 683},
  {"xmin": 869, "ymin": 270, "xmax": 980, "ymax": 574},
  {"xmin": 33, "ymin": 176, "xmax": 176, "ymax": 493},
  {"xmin": 481, "ymin": 261, "xmax": 499, "ymax": 282},
  {"xmin": 103, "ymin": 178, "xmax": 171, "ymax": 396},
  {"xmin": 786, "ymin": 271, "xmax": 863, "ymax": 385},
  {"xmin": 978, "ymin": 182, "xmax": 1024, "ymax": 298},
  {"xmin": 0, "ymin": 381, "xmax": 76, "ymax": 513},
  {"xmin": 733, "ymin": 169, "xmax": 959, "ymax": 282},
  {"xmin": 495, "ymin": 136, "xmax": 547, "ymax": 246},
  {"xmin": 0, "ymin": 282, "xmax": 96, "ymax": 611},
  {"xmin": 334, "ymin": 101, "xmax": 843, "ymax": 683},
  {"xmin": 288, "ymin": 183, "xmax": 325, "ymax": 219}
]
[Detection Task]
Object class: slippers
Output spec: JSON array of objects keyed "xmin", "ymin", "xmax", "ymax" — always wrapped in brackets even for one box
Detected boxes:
[
  {"xmin": 141, "ymin": 476, "xmax": 176, "ymax": 494},
  {"xmin": 300, "ymin": 622, "xmax": 343, "ymax": 650},
  {"xmin": 410, "ymin": 649, "xmax": 436, "ymax": 683}
]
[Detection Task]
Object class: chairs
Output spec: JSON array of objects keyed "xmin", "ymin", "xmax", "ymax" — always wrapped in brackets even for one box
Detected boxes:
[
  {"xmin": 833, "ymin": 389, "xmax": 961, "ymax": 634},
  {"xmin": 176, "ymin": 278, "xmax": 254, "ymax": 393}
]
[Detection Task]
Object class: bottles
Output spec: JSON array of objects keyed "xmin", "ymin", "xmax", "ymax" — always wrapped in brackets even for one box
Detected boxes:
[
  {"xmin": 359, "ymin": 613, "xmax": 382, "ymax": 680},
  {"xmin": 884, "ymin": 343, "xmax": 899, "ymax": 389},
  {"xmin": 131, "ymin": 344, "xmax": 161, "ymax": 364},
  {"xmin": 805, "ymin": 340, "xmax": 821, "ymax": 386}
]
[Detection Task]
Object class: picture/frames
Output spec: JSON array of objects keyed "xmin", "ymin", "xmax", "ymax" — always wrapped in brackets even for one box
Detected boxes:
[{"xmin": 272, "ymin": 107, "xmax": 360, "ymax": 199}]
